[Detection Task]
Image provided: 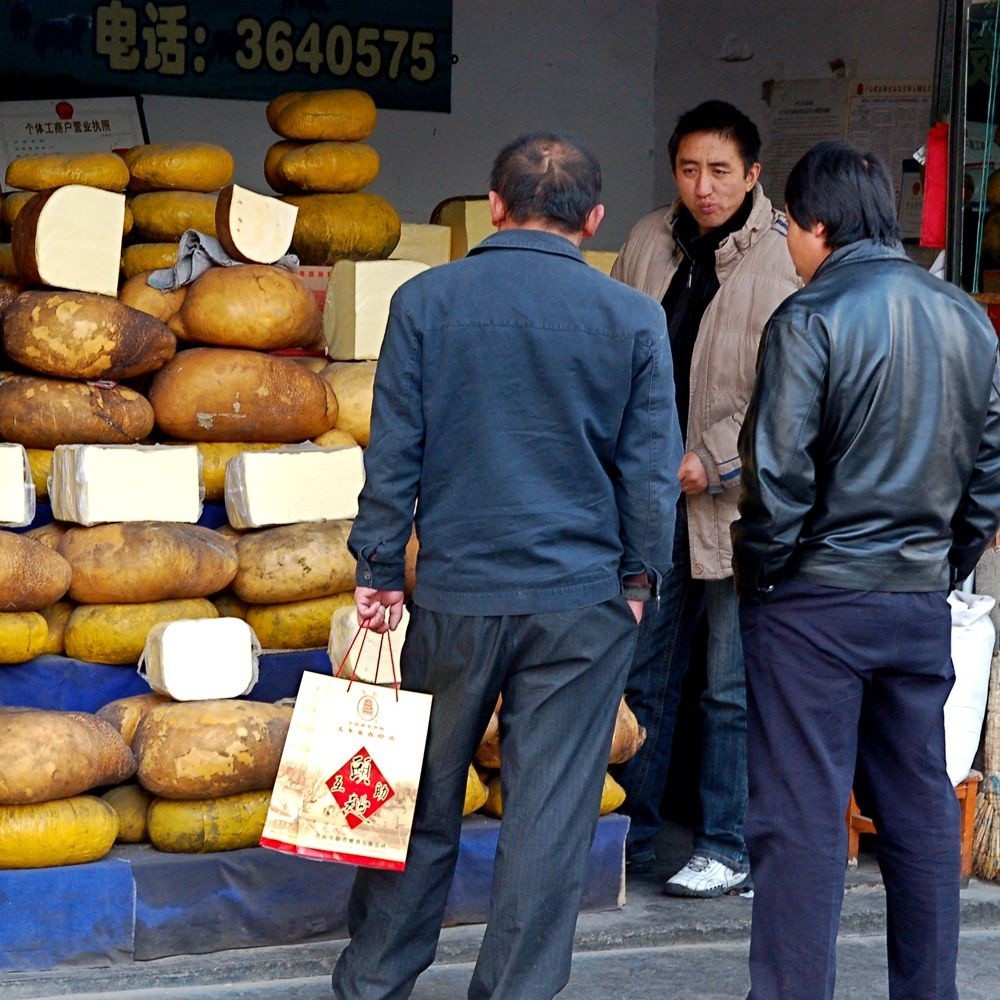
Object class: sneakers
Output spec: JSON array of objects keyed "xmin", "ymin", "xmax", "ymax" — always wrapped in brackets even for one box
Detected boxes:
[{"xmin": 664, "ymin": 851, "xmax": 750, "ymax": 898}]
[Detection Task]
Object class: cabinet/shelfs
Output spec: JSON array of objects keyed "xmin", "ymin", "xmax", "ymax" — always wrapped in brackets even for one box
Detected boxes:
[{"xmin": 0, "ymin": 426, "xmax": 632, "ymax": 971}]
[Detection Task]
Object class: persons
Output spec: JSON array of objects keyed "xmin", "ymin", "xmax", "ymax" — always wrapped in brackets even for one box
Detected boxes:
[
  {"xmin": 727, "ymin": 137, "xmax": 1000, "ymax": 1000},
  {"xmin": 610, "ymin": 99, "xmax": 804, "ymax": 900},
  {"xmin": 332, "ymin": 132, "xmax": 683, "ymax": 1000}
]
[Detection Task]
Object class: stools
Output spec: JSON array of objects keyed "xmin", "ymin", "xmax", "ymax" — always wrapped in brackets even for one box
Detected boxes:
[{"xmin": 844, "ymin": 765, "xmax": 983, "ymax": 889}]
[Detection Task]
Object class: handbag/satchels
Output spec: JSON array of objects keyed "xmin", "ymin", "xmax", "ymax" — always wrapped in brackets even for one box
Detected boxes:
[{"xmin": 259, "ymin": 616, "xmax": 433, "ymax": 873}]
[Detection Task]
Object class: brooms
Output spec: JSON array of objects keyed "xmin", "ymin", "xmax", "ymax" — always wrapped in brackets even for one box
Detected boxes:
[{"xmin": 970, "ymin": 655, "xmax": 1000, "ymax": 887}]
